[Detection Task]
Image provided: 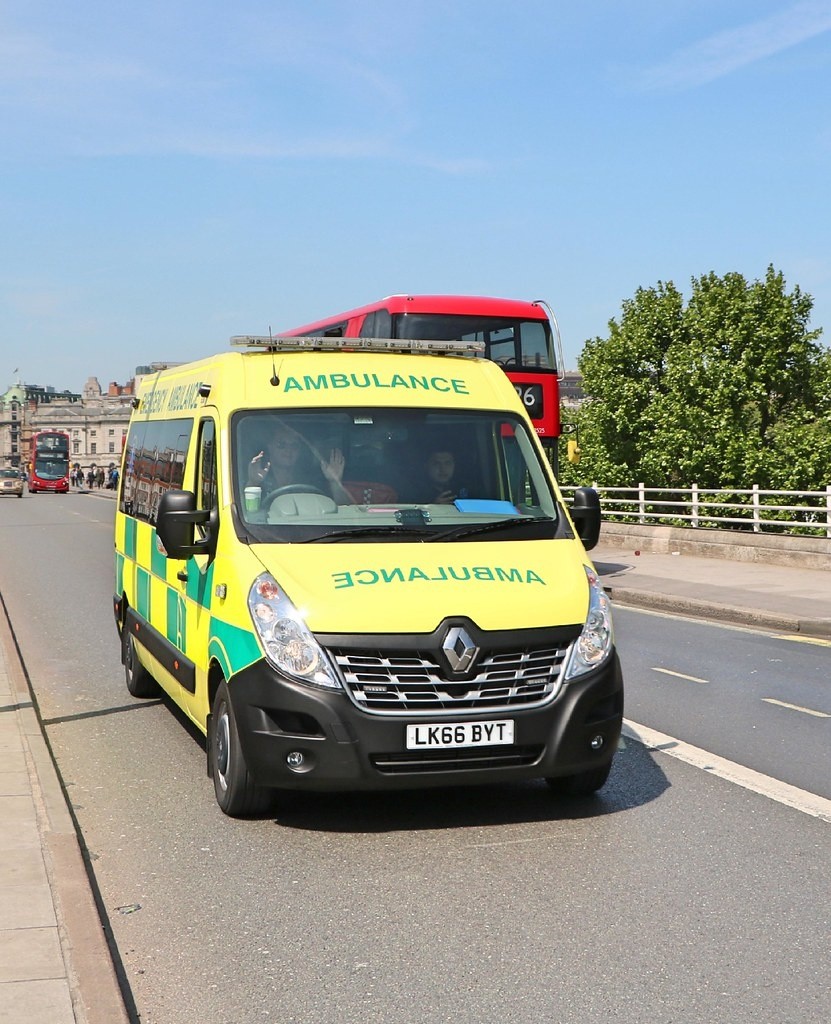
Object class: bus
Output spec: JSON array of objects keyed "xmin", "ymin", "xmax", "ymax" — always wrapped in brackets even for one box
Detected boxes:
[
  {"xmin": 28, "ymin": 430, "xmax": 70, "ymax": 494},
  {"xmin": 266, "ymin": 294, "xmax": 581, "ymax": 495}
]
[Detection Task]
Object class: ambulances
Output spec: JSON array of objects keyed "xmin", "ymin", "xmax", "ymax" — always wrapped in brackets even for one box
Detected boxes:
[{"xmin": 113, "ymin": 335, "xmax": 626, "ymax": 821}]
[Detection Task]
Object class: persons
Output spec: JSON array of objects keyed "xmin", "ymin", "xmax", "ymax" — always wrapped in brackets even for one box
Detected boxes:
[
  {"xmin": 242, "ymin": 427, "xmax": 350, "ymax": 506},
  {"xmin": 69, "ymin": 465, "xmax": 119, "ymax": 491},
  {"xmin": 398, "ymin": 448, "xmax": 475, "ymax": 506}
]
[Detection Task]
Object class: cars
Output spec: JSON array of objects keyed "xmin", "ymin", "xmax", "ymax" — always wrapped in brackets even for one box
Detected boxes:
[
  {"xmin": 20, "ymin": 472, "xmax": 27, "ymax": 482},
  {"xmin": 0, "ymin": 467, "xmax": 24, "ymax": 498}
]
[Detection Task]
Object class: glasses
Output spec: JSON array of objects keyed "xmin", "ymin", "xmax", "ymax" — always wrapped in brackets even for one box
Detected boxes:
[{"xmin": 272, "ymin": 438, "xmax": 300, "ymax": 451}]
